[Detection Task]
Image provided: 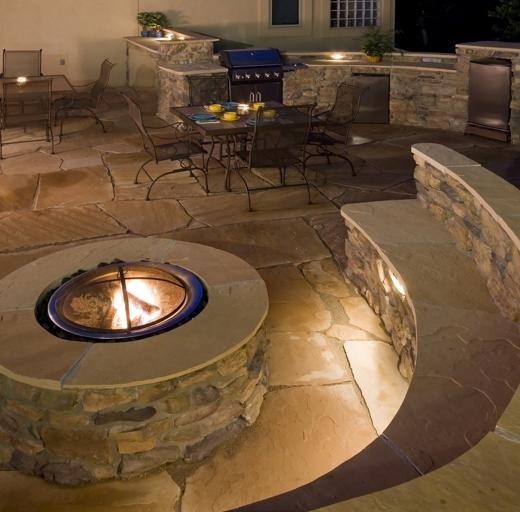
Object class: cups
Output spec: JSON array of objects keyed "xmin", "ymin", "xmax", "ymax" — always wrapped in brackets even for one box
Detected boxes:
[
  {"xmin": 253, "ymin": 102, "xmax": 266, "ymax": 109},
  {"xmin": 210, "ymin": 104, "xmax": 221, "ymax": 111},
  {"xmin": 223, "ymin": 112, "xmax": 237, "ymax": 120},
  {"xmin": 263, "ymin": 110, "xmax": 277, "ymax": 117}
]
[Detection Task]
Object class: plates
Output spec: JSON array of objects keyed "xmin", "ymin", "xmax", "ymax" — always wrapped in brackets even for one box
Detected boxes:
[
  {"xmin": 155, "ymin": 37, "xmax": 171, "ymax": 41},
  {"xmin": 220, "ymin": 116, "xmax": 240, "ymax": 121},
  {"xmin": 207, "ymin": 108, "xmax": 225, "ymax": 113},
  {"xmin": 262, "ymin": 113, "xmax": 280, "ymax": 119}
]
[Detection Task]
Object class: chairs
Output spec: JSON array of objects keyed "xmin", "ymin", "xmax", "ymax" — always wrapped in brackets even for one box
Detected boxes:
[
  {"xmin": 121, "ymin": 75, "xmax": 370, "ymax": 213},
  {"xmin": 0, "ymin": 49, "xmax": 118, "ymax": 161}
]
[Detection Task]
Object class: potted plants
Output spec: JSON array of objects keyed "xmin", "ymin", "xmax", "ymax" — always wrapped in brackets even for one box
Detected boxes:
[
  {"xmin": 350, "ymin": 24, "xmax": 405, "ymax": 64},
  {"xmin": 136, "ymin": 11, "xmax": 171, "ymax": 38}
]
